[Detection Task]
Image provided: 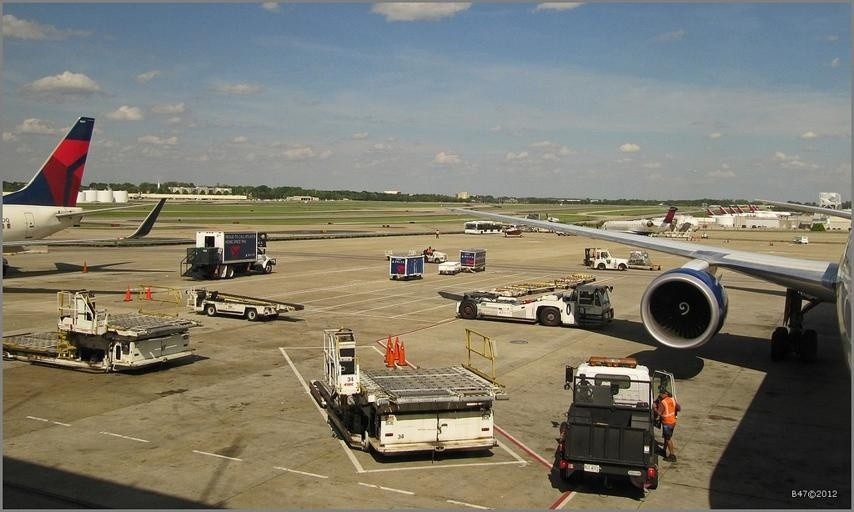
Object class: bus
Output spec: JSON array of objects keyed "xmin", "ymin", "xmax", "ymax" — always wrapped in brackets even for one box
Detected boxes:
[{"xmin": 462, "ymin": 221, "xmax": 502, "ymax": 234}]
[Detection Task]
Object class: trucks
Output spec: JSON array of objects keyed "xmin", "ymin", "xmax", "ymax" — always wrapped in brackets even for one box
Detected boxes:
[
  {"xmin": 180, "ymin": 230, "xmax": 275, "ymax": 279},
  {"xmin": 455, "ymin": 283, "xmax": 613, "ymax": 330},
  {"xmin": 556, "ymin": 354, "xmax": 677, "ymax": 491}
]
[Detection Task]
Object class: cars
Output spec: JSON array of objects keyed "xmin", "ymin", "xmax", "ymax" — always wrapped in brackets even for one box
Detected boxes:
[{"xmin": 583, "ymin": 248, "xmax": 627, "ymax": 270}]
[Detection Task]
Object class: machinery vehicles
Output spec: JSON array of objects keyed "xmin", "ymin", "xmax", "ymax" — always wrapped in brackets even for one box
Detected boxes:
[{"xmin": 184, "ymin": 287, "xmax": 304, "ymax": 321}]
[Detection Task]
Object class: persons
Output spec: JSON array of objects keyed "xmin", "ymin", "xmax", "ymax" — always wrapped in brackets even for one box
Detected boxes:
[{"xmin": 652, "ymin": 389, "xmax": 682, "ymax": 461}]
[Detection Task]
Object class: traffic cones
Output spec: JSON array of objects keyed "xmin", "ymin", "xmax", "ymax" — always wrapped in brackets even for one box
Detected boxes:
[
  {"xmin": 123, "ymin": 284, "xmax": 132, "ymax": 301},
  {"xmin": 384, "ymin": 335, "xmax": 406, "ymax": 367},
  {"xmin": 146, "ymin": 288, "xmax": 151, "ymax": 299},
  {"xmin": 83, "ymin": 259, "xmax": 87, "ymax": 273}
]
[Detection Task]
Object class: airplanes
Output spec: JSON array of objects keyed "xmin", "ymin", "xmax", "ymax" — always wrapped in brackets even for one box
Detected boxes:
[
  {"xmin": 0, "ymin": 114, "xmax": 167, "ymax": 278},
  {"xmin": 448, "ymin": 207, "xmax": 853, "ymax": 384}
]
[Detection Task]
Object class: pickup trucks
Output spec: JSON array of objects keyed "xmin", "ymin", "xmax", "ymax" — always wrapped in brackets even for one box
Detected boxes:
[{"xmin": 421, "ymin": 251, "xmax": 447, "ymax": 263}]
